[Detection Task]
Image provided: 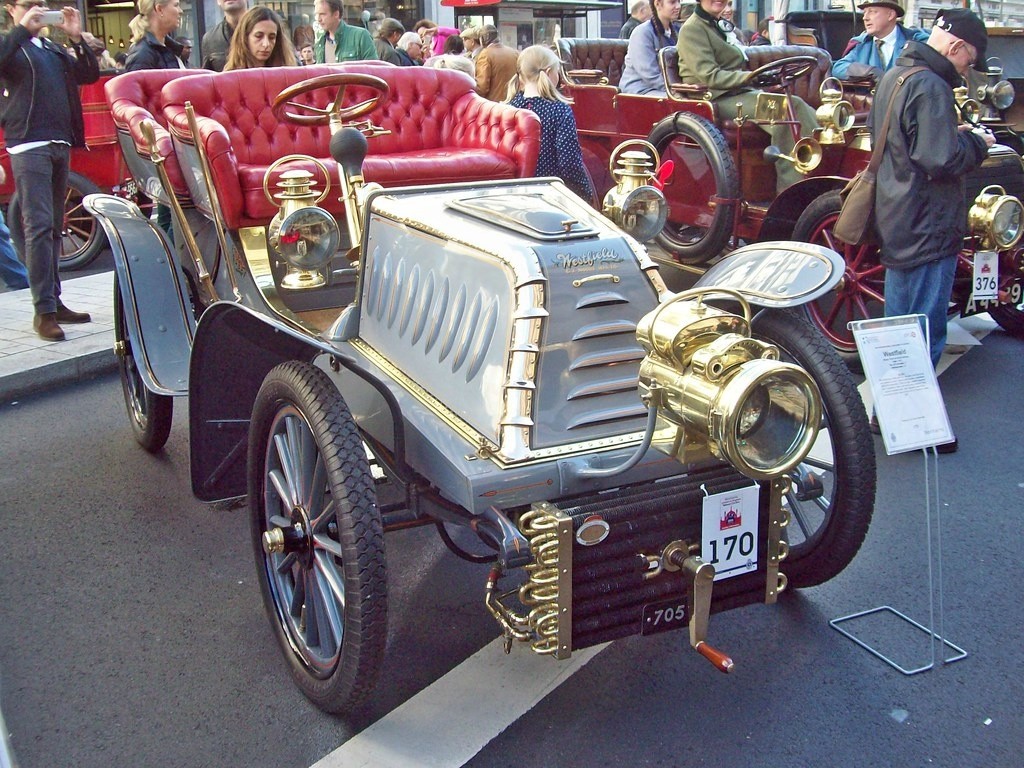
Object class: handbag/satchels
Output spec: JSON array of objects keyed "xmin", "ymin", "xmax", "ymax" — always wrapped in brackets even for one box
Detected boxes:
[{"xmin": 833, "ymin": 170, "xmax": 877, "ymax": 245}]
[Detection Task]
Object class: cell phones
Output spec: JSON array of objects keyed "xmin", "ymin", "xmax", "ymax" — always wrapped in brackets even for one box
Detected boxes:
[{"xmin": 38, "ymin": 11, "xmax": 63, "ymax": 25}]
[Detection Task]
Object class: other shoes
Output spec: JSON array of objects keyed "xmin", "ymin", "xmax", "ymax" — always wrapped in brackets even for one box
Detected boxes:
[
  {"xmin": 870, "ymin": 416, "xmax": 882, "ymax": 434},
  {"xmin": 920, "ymin": 438, "xmax": 959, "ymax": 454},
  {"xmin": 33, "ymin": 312, "xmax": 66, "ymax": 341},
  {"xmin": 55, "ymin": 302, "xmax": 91, "ymax": 324}
]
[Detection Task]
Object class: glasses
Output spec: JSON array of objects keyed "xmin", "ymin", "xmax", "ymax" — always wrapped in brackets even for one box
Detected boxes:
[
  {"xmin": 12, "ymin": 0, "xmax": 48, "ymax": 11},
  {"xmin": 414, "ymin": 42, "xmax": 423, "ymax": 50},
  {"xmin": 950, "ymin": 41, "xmax": 975, "ymax": 71}
]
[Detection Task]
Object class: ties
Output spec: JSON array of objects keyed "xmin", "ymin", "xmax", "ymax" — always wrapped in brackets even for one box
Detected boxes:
[{"xmin": 875, "ymin": 39, "xmax": 886, "ymax": 71}]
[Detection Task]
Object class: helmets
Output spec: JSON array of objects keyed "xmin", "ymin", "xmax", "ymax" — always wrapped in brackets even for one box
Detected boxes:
[{"xmin": 477, "ymin": 24, "xmax": 498, "ymax": 46}]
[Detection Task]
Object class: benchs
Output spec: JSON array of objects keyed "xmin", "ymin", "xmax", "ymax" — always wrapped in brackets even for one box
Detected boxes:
[
  {"xmin": 659, "ymin": 46, "xmax": 872, "ymax": 202},
  {"xmin": 102, "ymin": 58, "xmax": 398, "ymax": 197},
  {"xmin": 164, "ymin": 64, "xmax": 540, "ymax": 269},
  {"xmin": 558, "ymin": 38, "xmax": 633, "ymax": 84},
  {"xmin": 783, "ymin": 10, "xmax": 903, "ymax": 61}
]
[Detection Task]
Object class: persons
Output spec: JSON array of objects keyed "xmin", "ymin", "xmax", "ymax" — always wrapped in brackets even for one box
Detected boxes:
[
  {"xmin": 618, "ymin": 0, "xmax": 684, "ymax": 99},
  {"xmin": 395, "ymin": 19, "xmax": 482, "ymax": 78},
  {"xmin": 356, "ymin": 10, "xmax": 370, "ymax": 30},
  {"xmin": 299, "ymin": 42, "xmax": 316, "ymax": 65},
  {"xmin": 373, "ymin": 18, "xmax": 405, "ymax": 66},
  {"xmin": 500, "ymin": 45, "xmax": 594, "ymax": 208},
  {"xmin": 475, "ymin": 24, "xmax": 519, "ymax": 103},
  {"xmin": 0, "ymin": 0, "xmax": 100, "ymax": 342},
  {"xmin": 175, "ymin": 36, "xmax": 192, "ymax": 68},
  {"xmin": 313, "ymin": 0, "xmax": 379, "ymax": 64},
  {"xmin": 618, "ymin": 0, "xmax": 652, "ymax": 39},
  {"xmin": 201, "ymin": 0, "xmax": 303, "ymax": 72},
  {"xmin": 222, "ymin": 4, "xmax": 298, "ymax": 71},
  {"xmin": 676, "ymin": 0, "xmax": 820, "ymax": 195},
  {"xmin": 832, "ymin": 0, "xmax": 931, "ymax": 84},
  {"xmin": 81, "ymin": 31, "xmax": 128, "ymax": 70},
  {"xmin": 125, "ymin": 0, "xmax": 187, "ymax": 234},
  {"xmin": 293, "ymin": 14, "xmax": 315, "ymax": 50},
  {"xmin": 866, "ymin": 7, "xmax": 996, "ymax": 454}
]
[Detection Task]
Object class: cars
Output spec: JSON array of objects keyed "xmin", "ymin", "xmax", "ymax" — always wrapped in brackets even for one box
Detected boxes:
[
  {"xmin": 83, "ymin": 58, "xmax": 877, "ymax": 717},
  {"xmin": 550, "ymin": 34, "xmax": 1023, "ymax": 372},
  {"xmin": 0, "ymin": 69, "xmax": 139, "ymax": 272}
]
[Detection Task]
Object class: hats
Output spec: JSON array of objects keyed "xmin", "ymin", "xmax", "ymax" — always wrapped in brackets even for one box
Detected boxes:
[
  {"xmin": 857, "ymin": 0, "xmax": 905, "ymax": 17},
  {"xmin": 381, "ymin": 18, "xmax": 404, "ymax": 35},
  {"xmin": 934, "ymin": 8, "xmax": 991, "ymax": 74},
  {"xmin": 458, "ymin": 28, "xmax": 480, "ymax": 40}
]
